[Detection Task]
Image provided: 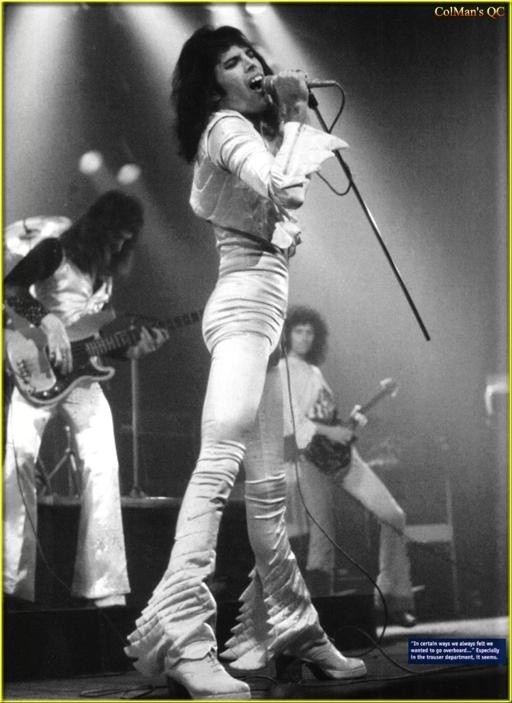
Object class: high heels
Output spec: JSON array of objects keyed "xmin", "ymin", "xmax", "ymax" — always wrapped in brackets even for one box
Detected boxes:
[
  {"xmin": 166, "ymin": 648, "xmax": 250, "ymax": 700},
  {"xmin": 274, "ymin": 631, "xmax": 367, "ymax": 682}
]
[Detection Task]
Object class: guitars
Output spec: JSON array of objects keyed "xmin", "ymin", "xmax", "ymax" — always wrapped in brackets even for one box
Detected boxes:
[
  {"xmin": 7, "ymin": 306, "xmax": 207, "ymax": 406},
  {"xmin": 302, "ymin": 379, "xmax": 400, "ymax": 476}
]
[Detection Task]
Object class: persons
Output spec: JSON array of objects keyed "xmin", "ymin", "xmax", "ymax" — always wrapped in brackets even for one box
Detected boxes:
[
  {"xmin": 276, "ymin": 304, "xmax": 418, "ymax": 630},
  {"xmin": 3, "ymin": 189, "xmax": 170, "ymax": 610},
  {"xmin": 123, "ymin": 25, "xmax": 369, "ymax": 698}
]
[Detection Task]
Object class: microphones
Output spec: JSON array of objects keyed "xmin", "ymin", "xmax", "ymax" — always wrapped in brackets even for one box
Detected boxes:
[{"xmin": 262, "ymin": 72, "xmax": 332, "ymax": 91}]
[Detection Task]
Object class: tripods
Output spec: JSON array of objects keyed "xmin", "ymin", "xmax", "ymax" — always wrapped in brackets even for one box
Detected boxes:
[{"xmin": 40, "ymin": 426, "xmax": 85, "ymax": 498}]
